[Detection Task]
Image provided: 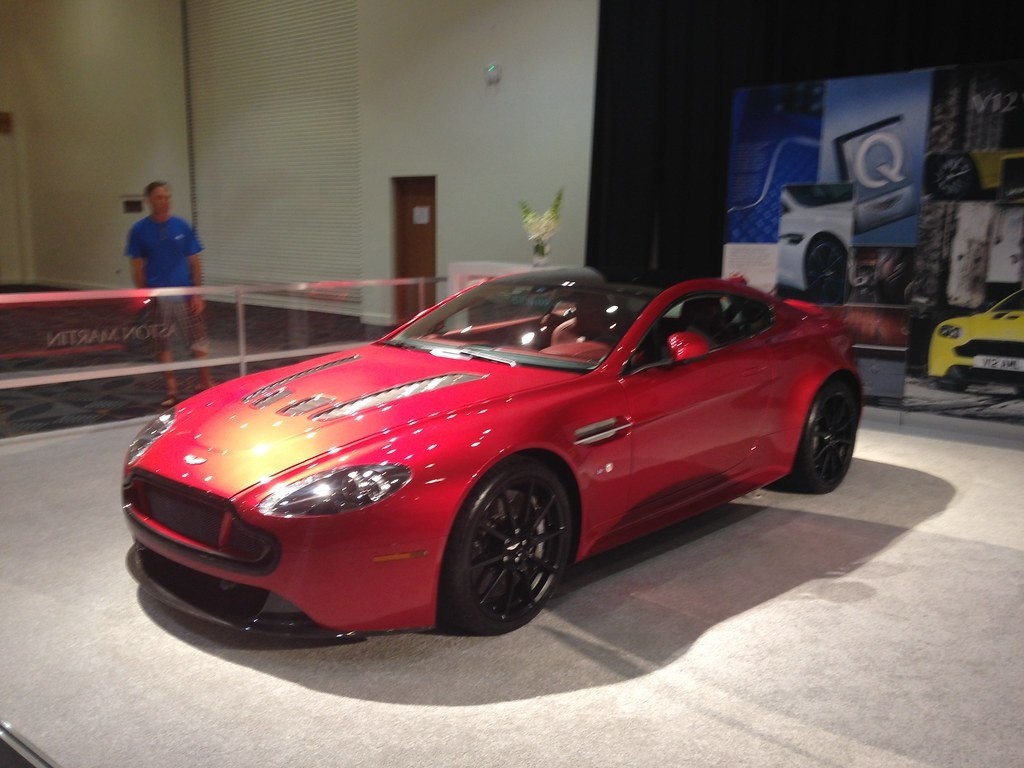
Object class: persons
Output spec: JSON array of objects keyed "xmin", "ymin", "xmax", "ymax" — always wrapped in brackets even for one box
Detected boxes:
[{"xmin": 125, "ymin": 181, "xmax": 216, "ymax": 408}]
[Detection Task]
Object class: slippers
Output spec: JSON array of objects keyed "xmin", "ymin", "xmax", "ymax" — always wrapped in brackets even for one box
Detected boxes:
[{"xmin": 162, "ymin": 395, "xmax": 176, "ymax": 409}]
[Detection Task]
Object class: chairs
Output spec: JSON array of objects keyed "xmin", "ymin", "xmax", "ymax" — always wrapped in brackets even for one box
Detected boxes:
[{"xmin": 662, "ymin": 297, "xmax": 732, "ymax": 348}]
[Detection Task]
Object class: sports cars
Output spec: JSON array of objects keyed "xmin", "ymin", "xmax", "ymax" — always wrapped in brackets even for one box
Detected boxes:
[
  {"xmin": 121, "ymin": 262, "xmax": 869, "ymax": 641},
  {"xmin": 926, "ymin": 282, "xmax": 1024, "ymax": 398}
]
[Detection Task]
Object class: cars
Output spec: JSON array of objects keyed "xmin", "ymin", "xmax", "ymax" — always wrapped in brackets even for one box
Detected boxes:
[
  {"xmin": 923, "ymin": 142, "xmax": 1024, "ymax": 206},
  {"xmin": 772, "ymin": 178, "xmax": 854, "ymax": 308}
]
